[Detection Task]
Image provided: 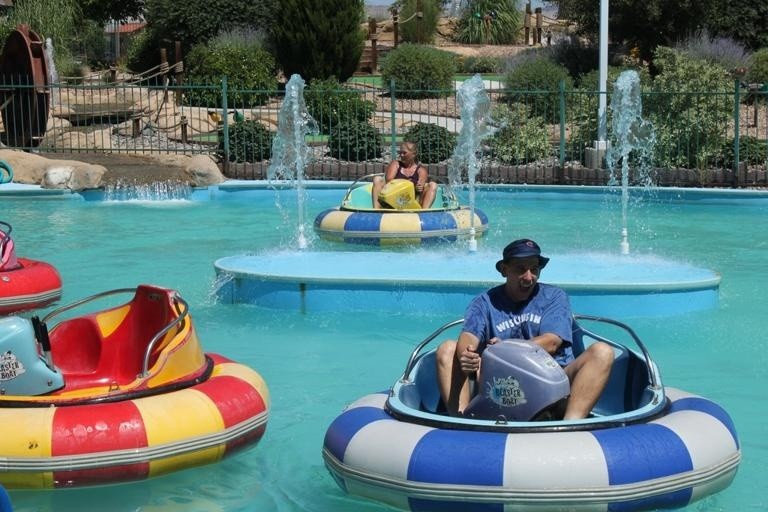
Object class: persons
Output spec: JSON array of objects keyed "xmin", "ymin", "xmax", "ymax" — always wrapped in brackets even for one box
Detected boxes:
[
  {"xmin": 433, "ymin": 239, "xmax": 614, "ymax": 424},
  {"xmin": 370, "ymin": 141, "xmax": 438, "ymax": 210}
]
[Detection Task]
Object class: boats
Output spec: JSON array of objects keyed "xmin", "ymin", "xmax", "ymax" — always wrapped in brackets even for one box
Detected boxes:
[
  {"xmin": 313, "ymin": 171, "xmax": 489, "ymax": 247},
  {"xmin": 0, "ymin": 218, "xmax": 62, "ymax": 322},
  {"xmin": 0, "ymin": 283, "xmax": 271, "ymax": 493},
  {"xmin": 323, "ymin": 313, "xmax": 740, "ymax": 511}
]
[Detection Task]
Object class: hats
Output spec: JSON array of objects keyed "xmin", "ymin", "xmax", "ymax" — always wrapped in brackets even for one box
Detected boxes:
[{"xmin": 496, "ymin": 238, "xmax": 549, "ymax": 272}]
